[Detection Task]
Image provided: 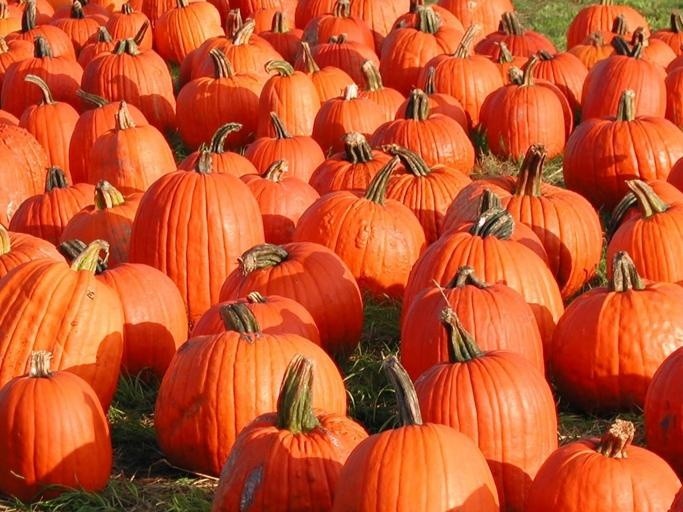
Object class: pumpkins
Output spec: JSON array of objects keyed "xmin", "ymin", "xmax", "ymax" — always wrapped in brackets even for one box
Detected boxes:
[{"xmin": 0, "ymin": 0, "xmax": 683, "ymax": 512}]
